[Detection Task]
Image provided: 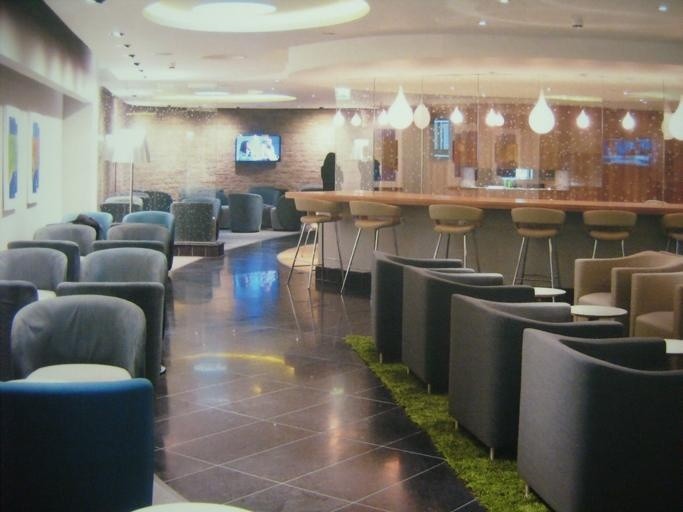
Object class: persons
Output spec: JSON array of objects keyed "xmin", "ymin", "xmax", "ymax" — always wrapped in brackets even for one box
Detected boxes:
[
  {"xmin": 321, "ymin": 152, "xmax": 344, "ymax": 191},
  {"xmin": 358, "ymin": 146, "xmax": 381, "ymax": 192},
  {"xmin": 238, "ymin": 138, "xmax": 253, "ymax": 160}
]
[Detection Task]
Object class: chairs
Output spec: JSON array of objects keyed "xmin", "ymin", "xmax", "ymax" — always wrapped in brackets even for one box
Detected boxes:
[
  {"xmin": 510, "ymin": 208, "xmax": 566, "ymax": 288},
  {"xmin": 660, "ymin": 213, "xmax": 682, "ymax": 254},
  {"xmin": 284, "ymin": 192, "xmax": 346, "ymax": 289},
  {"xmin": 428, "ymin": 205, "xmax": 484, "ymax": 269},
  {"xmin": 341, "ymin": 202, "xmax": 405, "ymax": 291},
  {"xmin": 582, "ymin": 210, "xmax": 636, "ymax": 259}
]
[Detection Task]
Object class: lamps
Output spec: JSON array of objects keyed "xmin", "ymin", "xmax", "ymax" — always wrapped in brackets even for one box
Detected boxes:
[
  {"xmin": 103, "ymin": 133, "xmax": 120, "ymax": 195},
  {"xmin": 108, "ymin": 127, "xmax": 149, "ymax": 212}
]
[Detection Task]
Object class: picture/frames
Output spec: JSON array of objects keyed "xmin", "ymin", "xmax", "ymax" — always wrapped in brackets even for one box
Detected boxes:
[
  {"xmin": 26, "ymin": 111, "xmax": 43, "ymax": 206},
  {"xmin": 3, "ymin": 100, "xmax": 27, "ymax": 212}
]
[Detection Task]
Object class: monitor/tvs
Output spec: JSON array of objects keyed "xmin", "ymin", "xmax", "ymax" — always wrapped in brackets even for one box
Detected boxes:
[
  {"xmin": 600, "ymin": 138, "xmax": 655, "ymax": 167},
  {"xmin": 234, "ymin": 132, "xmax": 282, "ymax": 163}
]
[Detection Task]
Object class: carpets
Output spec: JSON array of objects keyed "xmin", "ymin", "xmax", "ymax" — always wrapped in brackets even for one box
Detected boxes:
[{"xmin": 344, "ymin": 336, "xmax": 553, "ymax": 511}]
